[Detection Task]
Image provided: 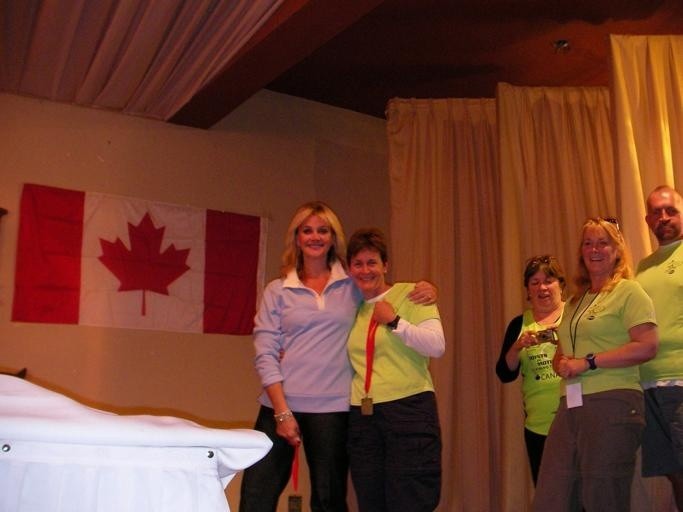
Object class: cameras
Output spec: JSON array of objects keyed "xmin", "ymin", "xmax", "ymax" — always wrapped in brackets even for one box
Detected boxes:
[{"xmin": 534, "ymin": 328, "xmax": 555, "ymax": 345}]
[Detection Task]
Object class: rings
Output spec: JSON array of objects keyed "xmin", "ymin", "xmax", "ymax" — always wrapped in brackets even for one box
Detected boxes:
[{"xmin": 427, "ymin": 295, "xmax": 431, "ymax": 299}]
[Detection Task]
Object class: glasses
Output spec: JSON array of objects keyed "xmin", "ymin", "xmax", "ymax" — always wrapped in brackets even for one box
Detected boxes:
[
  {"xmin": 525, "ymin": 255, "xmax": 558, "ymax": 267},
  {"xmin": 604, "ymin": 216, "xmax": 620, "ymax": 231}
]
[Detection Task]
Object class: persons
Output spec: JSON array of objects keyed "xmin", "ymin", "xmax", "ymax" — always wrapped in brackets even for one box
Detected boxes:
[
  {"xmin": 632, "ymin": 184, "xmax": 682, "ymax": 510},
  {"xmin": 494, "ymin": 254, "xmax": 567, "ymax": 487},
  {"xmin": 236, "ymin": 203, "xmax": 438, "ymax": 511},
  {"xmin": 343, "ymin": 226, "xmax": 447, "ymax": 509},
  {"xmin": 529, "ymin": 214, "xmax": 658, "ymax": 511}
]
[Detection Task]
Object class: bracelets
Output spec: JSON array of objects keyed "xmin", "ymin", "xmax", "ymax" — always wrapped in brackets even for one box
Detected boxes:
[
  {"xmin": 273, "ymin": 408, "xmax": 293, "ymax": 424},
  {"xmin": 388, "ymin": 314, "xmax": 401, "ymax": 327}
]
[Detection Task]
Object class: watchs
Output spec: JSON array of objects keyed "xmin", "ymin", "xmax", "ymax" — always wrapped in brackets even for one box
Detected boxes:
[{"xmin": 586, "ymin": 351, "xmax": 599, "ymax": 371}]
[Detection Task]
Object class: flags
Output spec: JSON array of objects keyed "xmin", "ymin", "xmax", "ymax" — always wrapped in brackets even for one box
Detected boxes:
[{"xmin": 10, "ymin": 181, "xmax": 269, "ymax": 336}]
